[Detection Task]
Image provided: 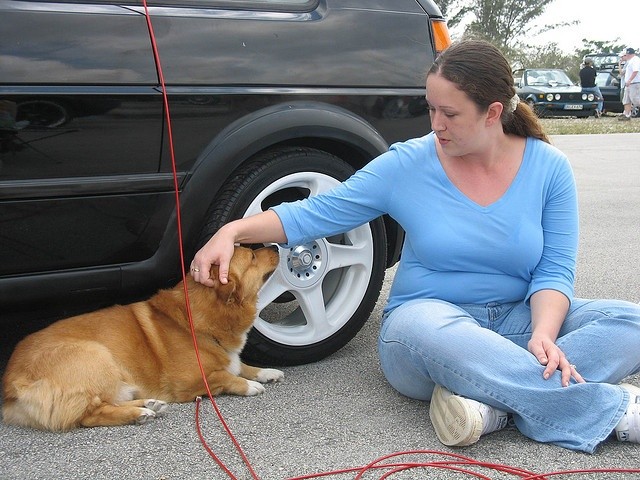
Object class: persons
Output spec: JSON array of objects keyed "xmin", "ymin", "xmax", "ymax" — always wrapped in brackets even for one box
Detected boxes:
[
  {"xmin": 618, "ymin": 47, "xmax": 640, "ymax": 120},
  {"xmin": 617, "ymin": 51, "xmax": 627, "ymax": 102},
  {"xmin": 191, "ymin": 38, "xmax": 640, "ymax": 447},
  {"xmin": 579, "ymin": 58, "xmax": 604, "ymax": 118}
]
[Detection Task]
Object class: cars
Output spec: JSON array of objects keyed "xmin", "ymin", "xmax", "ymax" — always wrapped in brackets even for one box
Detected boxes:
[{"xmin": 512, "ymin": 68, "xmax": 598, "ymax": 118}]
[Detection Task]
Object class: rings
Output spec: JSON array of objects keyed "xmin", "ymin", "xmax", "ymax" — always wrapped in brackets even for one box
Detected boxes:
[
  {"xmin": 192, "ymin": 269, "xmax": 200, "ymax": 272},
  {"xmin": 569, "ymin": 364, "xmax": 576, "ymax": 368}
]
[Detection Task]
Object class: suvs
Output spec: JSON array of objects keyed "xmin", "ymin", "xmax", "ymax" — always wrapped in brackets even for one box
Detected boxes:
[
  {"xmin": 0, "ymin": 1, "xmax": 452, "ymax": 368},
  {"xmin": 579, "ymin": 52, "xmax": 640, "ymax": 117}
]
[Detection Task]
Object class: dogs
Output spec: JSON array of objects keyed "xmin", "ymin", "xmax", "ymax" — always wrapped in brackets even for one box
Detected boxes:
[{"xmin": 0, "ymin": 244, "xmax": 285, "ymax": 434}]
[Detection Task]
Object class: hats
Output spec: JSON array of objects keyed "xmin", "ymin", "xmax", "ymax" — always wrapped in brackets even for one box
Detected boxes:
[{"xmin": 626, "ymin": 48, "xmax": 635, "ymax": 54}]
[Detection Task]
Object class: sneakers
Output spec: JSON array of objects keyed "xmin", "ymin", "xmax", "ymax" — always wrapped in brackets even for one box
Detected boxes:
[
  {"xmin": 614, "ymin": 382, "xmax": 640, "ymax": 444},
  {"xmin": 429, "ymin": 382, "xmax": 509, "ymax": 447},
  {"xmin": 618, "ymin": 114, "xmax": 631, "ymax": 121},
  {"xmin": 594, "ymin": 108, "xmax": 600, "ymax": 118}
]
